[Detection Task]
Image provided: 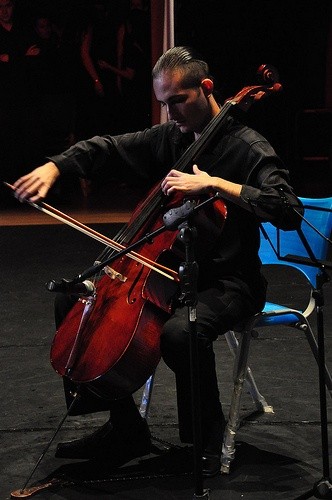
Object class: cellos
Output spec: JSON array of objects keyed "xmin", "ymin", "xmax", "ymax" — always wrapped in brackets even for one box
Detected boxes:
[{"xmin": 20, "ymin": 63, "xmax": 282, "ymax": 495}]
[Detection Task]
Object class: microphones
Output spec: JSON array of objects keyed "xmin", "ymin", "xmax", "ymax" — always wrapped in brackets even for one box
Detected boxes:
[{"xmin": 43, "ymin": 279, "xmax": 95, "ymax": 298}]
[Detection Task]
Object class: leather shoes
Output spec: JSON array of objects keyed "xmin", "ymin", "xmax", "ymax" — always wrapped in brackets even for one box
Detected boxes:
[
  {"xmin": 57, "ymin": 417, "xmax": 152, "ymax": 456},
  {"xmin": 196, "ymin": 439, "xmax": 223, "ymax": 478}
]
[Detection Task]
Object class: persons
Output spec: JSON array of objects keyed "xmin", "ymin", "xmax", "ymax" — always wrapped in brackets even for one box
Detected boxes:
[{"xmin": 14, "ymin": 48, "xmax": 304, "ymax": 477}]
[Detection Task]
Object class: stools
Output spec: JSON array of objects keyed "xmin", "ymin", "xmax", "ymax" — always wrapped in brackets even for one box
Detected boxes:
[{"xmin": 135, "ymin": 189, "xmax": 332, "ymax": 478}]
